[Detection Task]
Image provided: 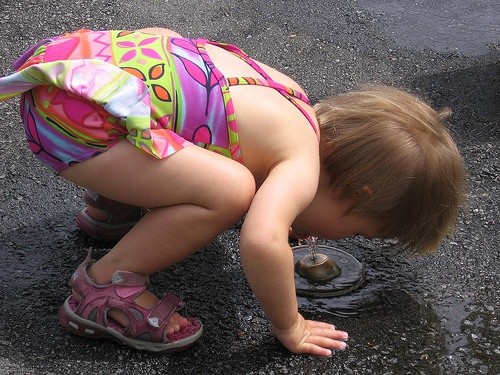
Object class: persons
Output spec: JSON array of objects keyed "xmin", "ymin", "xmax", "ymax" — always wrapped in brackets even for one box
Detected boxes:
[{"xmin": 11, "ymin": 30, "xmax": 467, "ymax": 357}]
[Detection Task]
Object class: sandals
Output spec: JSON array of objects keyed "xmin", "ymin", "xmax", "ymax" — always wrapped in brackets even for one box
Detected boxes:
[
  {"xmin": 75, "ymin": 188, "xmax": 148, "ymax": 242},
  {"xmin": 59, "ymin": 247, "xmax": 203, "ymax": 354}
]
